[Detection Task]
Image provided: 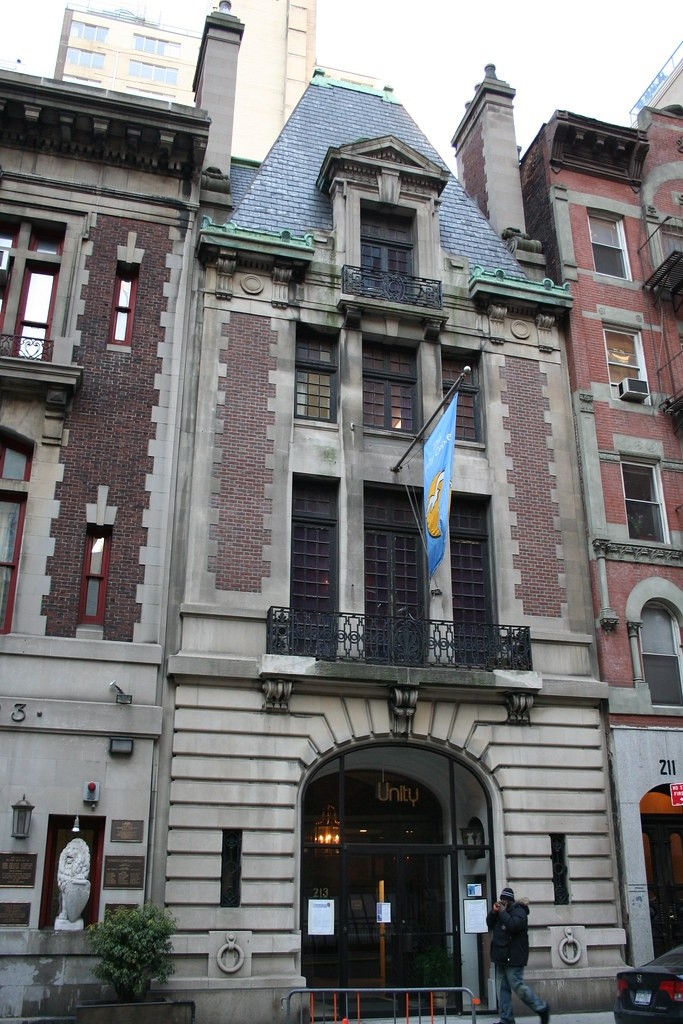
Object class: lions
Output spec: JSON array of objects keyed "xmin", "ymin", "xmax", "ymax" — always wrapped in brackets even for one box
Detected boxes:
[{"xmin": 56, "ymin": 837, "xmax": 91, "ymax": 923}]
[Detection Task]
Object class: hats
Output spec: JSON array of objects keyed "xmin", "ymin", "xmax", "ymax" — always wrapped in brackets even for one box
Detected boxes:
[{"xmin": 500, "ymin": 887, "xmax": 515, "ymax": 902}]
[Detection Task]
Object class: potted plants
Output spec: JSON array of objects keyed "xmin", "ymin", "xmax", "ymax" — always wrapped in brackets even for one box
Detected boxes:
[
  {"xmin": 415, "ymin": 945, "xmax": 465, "ymax": 1010},
  {"xmin": 73, "ymin": 898, "xmax": 173, "ymax": 1024}
]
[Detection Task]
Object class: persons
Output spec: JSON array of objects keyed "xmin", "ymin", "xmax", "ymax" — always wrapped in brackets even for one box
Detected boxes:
[{"xmin": 486, "ymin": 888, "xmax": 550, "ymax": 1024}]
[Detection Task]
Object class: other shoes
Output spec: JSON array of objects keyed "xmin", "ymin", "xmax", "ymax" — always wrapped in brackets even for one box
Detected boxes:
[
  {"xmin": 537, "ymin": 1002, "xmax": 550, "ymax": 1024},
  {"xmin": 493, "ymin": 1019, "xmax": 516, "ymax": 1024}
]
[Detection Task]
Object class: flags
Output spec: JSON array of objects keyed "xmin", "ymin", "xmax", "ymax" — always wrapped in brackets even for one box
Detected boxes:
[{"xmin": 424, "ymin": 392, "xmax": 458, "ymax": 577}]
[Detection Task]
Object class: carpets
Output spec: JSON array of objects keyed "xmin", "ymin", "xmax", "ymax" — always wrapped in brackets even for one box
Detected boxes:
[{"xmin": 315, "ymin": 997, "xmax": 417, "ymax": 1011}]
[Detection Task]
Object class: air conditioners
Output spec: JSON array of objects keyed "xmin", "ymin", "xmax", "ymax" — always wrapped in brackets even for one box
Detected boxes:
[{"xmin": 618, "ymin": 377, "xmax": 649, "ymax": 402}]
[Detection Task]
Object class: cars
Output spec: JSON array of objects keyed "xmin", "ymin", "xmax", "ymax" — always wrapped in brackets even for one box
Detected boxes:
[{"xmin": 613, "ymin": 945, "xmax": 683, "ymax": 1024}]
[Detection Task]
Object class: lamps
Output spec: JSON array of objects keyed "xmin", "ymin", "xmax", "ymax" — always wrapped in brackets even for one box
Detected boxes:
[
  {"xmin": 110, "ymin": 736, "xmax": 134, "ymax": 754},
  {"xmin": 71, "ymin": 812, "xmax": 80, "ymax": 832},
  {"xmin": 10, "ymin": 792, "xmax": 35, "ymax": 840},
  {"xmin": 310, "ymin": 800, "xmax": 340, "ymax": 855},
  {"xmin": 108, "ymin": 680, "xmax": 132, "ymax": 704}
]
